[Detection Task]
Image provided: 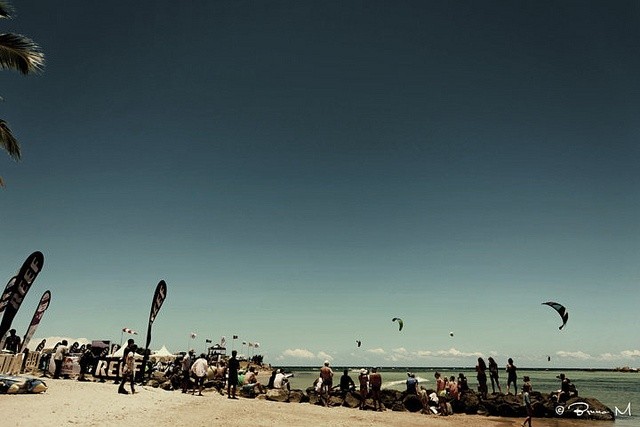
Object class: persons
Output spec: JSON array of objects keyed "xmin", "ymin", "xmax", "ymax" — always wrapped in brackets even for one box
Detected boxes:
[
  {"xmin": 118, "ymin": 339, "xmax": 137, "ymax": 395},
  {"xmin": 77, "ymin": 344, "xmax": 94, "ymax": 381},
  {"xmin": 369, "ymin": 366, "xmax": 382, "ymax": 411},
  {"xmin": 505, "ymin": 358, "xmax": 517, "ymax": 396},
  {"xmin": 192, "ymin": 353, "xmax": 207, "ymax": 395},
  {"xmin": 475, "ymin": 358, "xmax": 488, "ymax": 400},
  {"xmin": 341, "ymin": 370, "xmax": 356, "ymax": 393},
  {"xmin": 548, "ymin": 374, "xmax": 578, "ymax": 405},
  {"xmin": 268, "ymin": 368, "xmax": 290, "ymax": 389},
  {"xmin": 181, "ymin": 349, "xmax": 194, "ymax": 393},
  {"xmin": 521, "ymin": 385, "xmax": 534, "ymax": 427},
  {"xmin": 228, "ymin": 351, "xmax": 240, "ymax": 399},
  {"xmin": 359, "ymin": 368, "xmax": 368, "ymax": 410},
  {"xmin": 488, "ymin": 357, "xmax": 502, "ymax": 395},
  {"xmin": 306, "ymin": 360, "xmax": 333, "ymax": 407},
  {"xmin": 243, "ymin": 366, "xmax": 266, "ymax": 394},
  {"xmin": 521, "ymin": 376, "xmax": 533, "ymax": 392},
  {"xmin": 2, "ymin": 329, "xmax": 21, "ymax": 353},
  {"xmin": 403, "ymin": 372, "xmax": 468, "ymax": 416},
  {"xmin": 53, "ymin": 340, "xmax": 68, "ymax": 379}
]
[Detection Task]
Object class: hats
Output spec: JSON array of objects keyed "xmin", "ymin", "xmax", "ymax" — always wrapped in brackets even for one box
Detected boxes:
[
  {"xmin": 189, "ymin": 349, "xmax": 196, "ymax": 352},
  {"xmin": 324, "ymin": 360, "xmax": 329, "ymax": 364},
  {"xmin": 8, "ymin": 328, "xmax": 16, "ymax": 331},
  {"xmin": 360, "ymin": 369, "xmax": 367, "ymax": 373}
]
